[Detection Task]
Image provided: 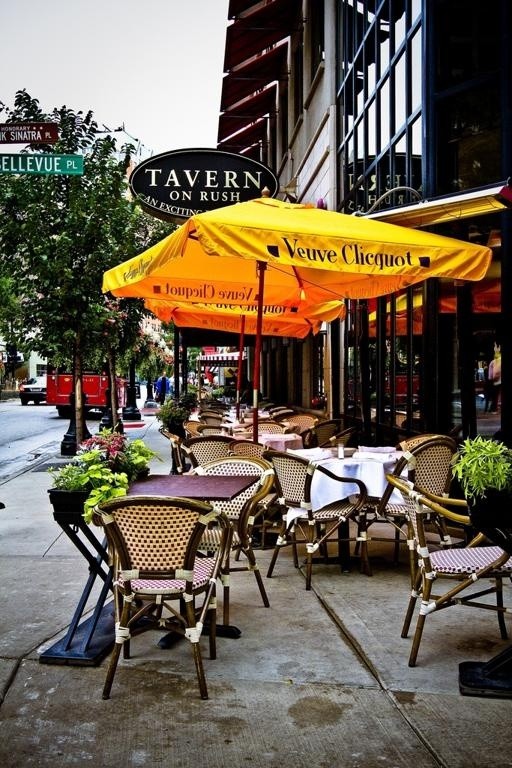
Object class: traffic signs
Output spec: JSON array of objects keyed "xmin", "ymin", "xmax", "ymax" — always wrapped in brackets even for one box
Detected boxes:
[
  {"xmin": 0, "ymin": 153, "xmax": 84, "ymax": 175},
  {"xmin": 0, "ymin": 122, "xmax": 59, "ymax": 144}
]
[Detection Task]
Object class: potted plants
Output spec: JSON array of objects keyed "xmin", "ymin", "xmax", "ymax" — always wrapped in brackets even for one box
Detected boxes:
[
  {"xmin": 46, "ymin": 423, "xmax": 165, "ymax": 527},
  {"xmin": 447, "ymin": 433, "xmax": 512, "ymax": 533}
]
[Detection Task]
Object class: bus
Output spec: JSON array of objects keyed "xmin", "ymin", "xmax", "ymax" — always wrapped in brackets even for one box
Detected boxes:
[
  {"xmin": 348, "ymin": 343, "xmax": 421, "ymax": 413},
  {"xmin": 46, "ymin": 350, "xmax": 128, "ymax": 420}
]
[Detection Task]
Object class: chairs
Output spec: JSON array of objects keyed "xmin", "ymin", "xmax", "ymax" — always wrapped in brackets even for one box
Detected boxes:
[
  {"xmin": 386, "ymin": 474, "xmax": 510, "ymax": 669},
  {"xmin": 90, "ymin": 494, "xmax": 241, "ymax": 700},
  {"xmin": 152, "ymin": 396, "xmax": 460, "ymax": 593}
]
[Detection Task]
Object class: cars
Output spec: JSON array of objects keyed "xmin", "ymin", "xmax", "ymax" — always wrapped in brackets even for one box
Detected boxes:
[
  {"xmin": 451, "ymin": 390, "xmax": 487, "ymax": 418},
  {"xmin": 19, "ymin": 376, "xmax": 47, "ymax": 405}
]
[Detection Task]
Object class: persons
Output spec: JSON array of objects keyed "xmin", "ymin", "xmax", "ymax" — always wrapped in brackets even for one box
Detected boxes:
[
  {"xmin": 241, "ymin": 380, "xmax": 262, "ymax": 404},
  {"xmin": 156, "ymin": 371, "xmax": 170, "ymax": 406},
  {"xmin": 168, "ymin": 372, "xmax": 181, "ymax": 396}
]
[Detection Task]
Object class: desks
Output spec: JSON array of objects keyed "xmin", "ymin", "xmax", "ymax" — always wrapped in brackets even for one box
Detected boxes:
[{"xmin": 125, "ymin": 474, "xmax": 262, "ymax": 502}]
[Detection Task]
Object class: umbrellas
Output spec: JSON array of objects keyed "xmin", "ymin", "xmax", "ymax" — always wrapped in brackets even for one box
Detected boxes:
[
  {"xmin": 102, "ymin": 186, "xmax": 494, "ymax": 444},
  {"xmin": 144, "ymin": 298, "xmax": 348, "ymax": 418}
]
